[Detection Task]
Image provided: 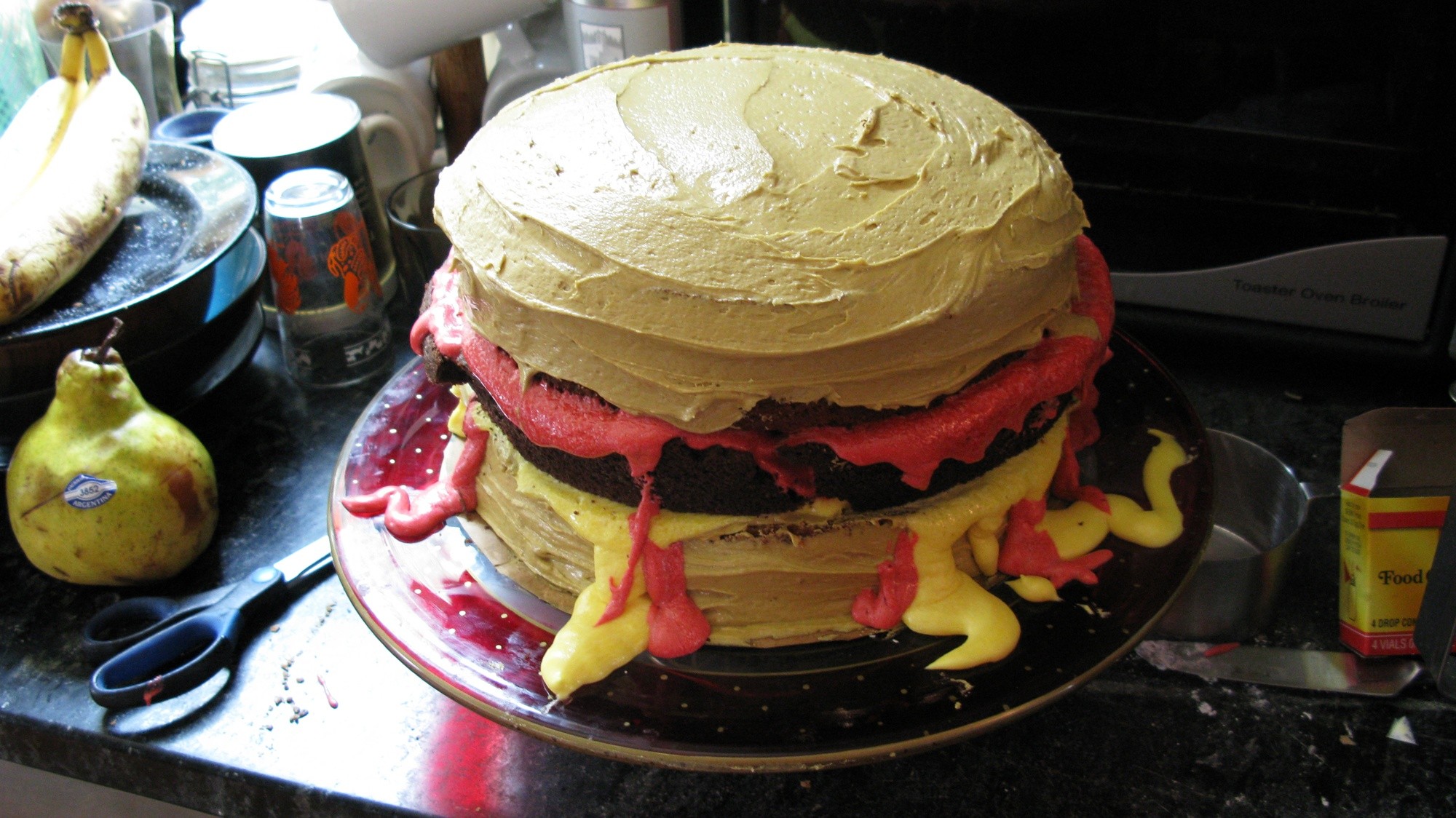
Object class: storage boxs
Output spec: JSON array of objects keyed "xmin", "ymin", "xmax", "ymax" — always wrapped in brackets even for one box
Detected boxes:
[{"xmin": 1338, "ymin": 404, "xmax": 1456, "ymax": 661}]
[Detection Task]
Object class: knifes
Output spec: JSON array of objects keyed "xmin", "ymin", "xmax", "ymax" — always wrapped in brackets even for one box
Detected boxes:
[{"xmin": 1134, "ymin": 639, "xmax": 1440, "ymax": 698}]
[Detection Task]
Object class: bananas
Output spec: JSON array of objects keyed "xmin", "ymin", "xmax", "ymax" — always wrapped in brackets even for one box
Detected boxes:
[{"xmin": 1, "ymin": 3, "xmax": 150, "ymax": 321}]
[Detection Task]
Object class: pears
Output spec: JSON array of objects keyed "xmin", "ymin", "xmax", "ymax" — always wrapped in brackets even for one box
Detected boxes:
[{"xmin": 6, "ymin": 320, "xmax": 215, "ymax": 585}]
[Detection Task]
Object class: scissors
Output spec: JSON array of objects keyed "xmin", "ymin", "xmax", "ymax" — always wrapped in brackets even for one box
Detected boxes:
[{"xmin": 83, "ymin": 532, "xmax": 334, "ymax": 709}]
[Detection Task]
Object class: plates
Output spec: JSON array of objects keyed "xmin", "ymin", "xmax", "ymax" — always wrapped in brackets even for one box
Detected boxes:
[{"xmin": 1, "ymin": 141, "xmax": 269, "ymax": 477}]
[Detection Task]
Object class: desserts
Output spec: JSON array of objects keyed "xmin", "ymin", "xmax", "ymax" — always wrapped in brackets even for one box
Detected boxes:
[{"xmin": 339, "ymin": 39, "xmax": 1194, "ymax": 703}]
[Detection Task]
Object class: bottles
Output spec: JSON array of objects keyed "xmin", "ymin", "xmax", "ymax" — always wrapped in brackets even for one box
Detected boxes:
[{"xmin": 174, "ymin": 0, "xmax": 310, "ymax": 113}]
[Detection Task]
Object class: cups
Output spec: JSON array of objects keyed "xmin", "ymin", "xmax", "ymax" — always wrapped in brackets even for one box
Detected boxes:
[
  {"xmin": 212, "ymin": 92, "xmax": 420, "ymax": 336},
  {"xmin": 384, "ymin": 165, "xmax": 452, "ymax": 324},
  {"xmin": 153, "ymin": 108, "xmax": 233, "ymax": 149},
  {"xmin": 312, "ymin": 0, "xmax": 685, "ymax": 193},
  {"xmin": 1140, "ymin": 428, "xmax": 1313, "ymax": 645},
  {"xmin": 36, "ymin": 1, "xmax": 184, "ymax": 141},
  {"xmin": 263, "ymin": 168, "xmax": 393, "ymax": 388}
]
